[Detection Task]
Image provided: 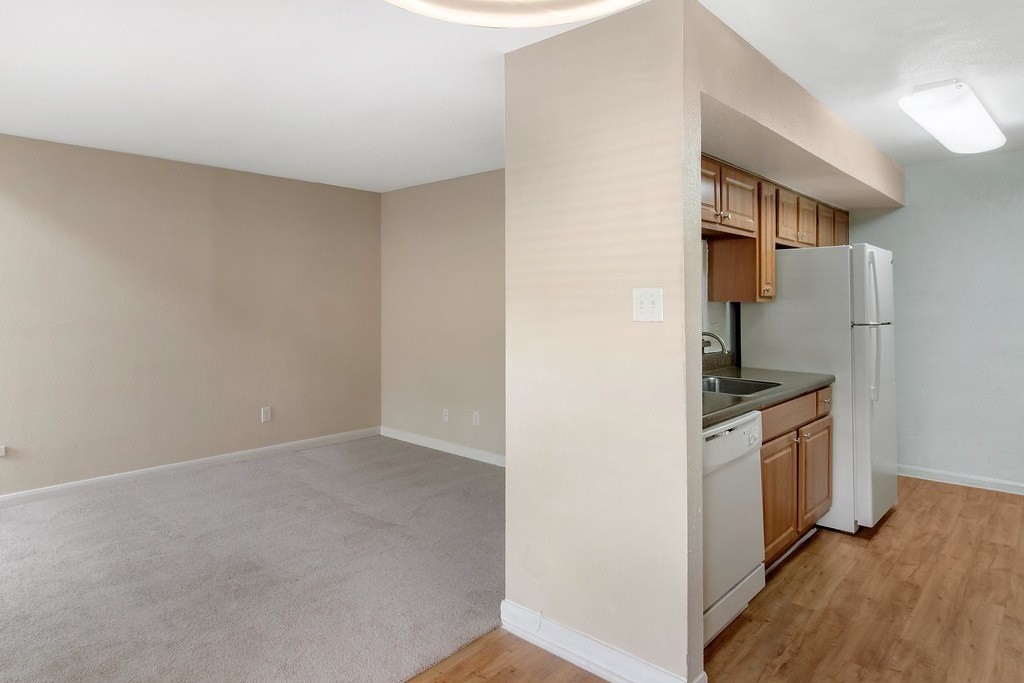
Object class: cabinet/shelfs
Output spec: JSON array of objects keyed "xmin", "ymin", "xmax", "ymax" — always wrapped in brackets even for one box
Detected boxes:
[
  {"xmin": 776, "ymin": 183, "xmax": 817, "ymax": 248},
  {"xmin": 708, "ymin": 177, "xmax": 776, "ymax": 303},
  {"xmin": 760, "ymin": 385, "xmax": 834, "ymax": 577},
  {"xmin": 817, "ymin": 200, "xmax": 849, "ymax": 247},
  {"xmin": 702, "ymin": 152, "xmax": 757, "ymax": 240}
]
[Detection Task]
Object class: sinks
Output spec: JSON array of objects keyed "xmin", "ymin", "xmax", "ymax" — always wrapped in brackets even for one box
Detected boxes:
[{"xmin": 703, "ymin": 377, "xmax": 782, "ymax": 395}]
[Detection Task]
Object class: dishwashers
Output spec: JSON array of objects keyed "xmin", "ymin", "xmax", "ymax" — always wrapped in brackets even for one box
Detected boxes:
[{"xmin": 702, "ymin": 409, "xmax": 766, "ymax": 652}]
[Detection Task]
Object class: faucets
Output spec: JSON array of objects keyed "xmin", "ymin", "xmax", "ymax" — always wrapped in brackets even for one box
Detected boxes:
[{"xmin": 702, "ymin": 333, "xmax": 728, "ymax": 356}]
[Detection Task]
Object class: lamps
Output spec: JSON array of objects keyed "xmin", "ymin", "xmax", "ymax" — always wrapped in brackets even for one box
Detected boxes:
[{"xmin": 897, "ymin": 78, "xmax": 1007, "ymax": 155}]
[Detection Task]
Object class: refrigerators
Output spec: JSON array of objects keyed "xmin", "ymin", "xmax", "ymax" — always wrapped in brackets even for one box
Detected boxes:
[{"xmin": 740, "ymin": 242, "xmax": 898, "ymax": 536}]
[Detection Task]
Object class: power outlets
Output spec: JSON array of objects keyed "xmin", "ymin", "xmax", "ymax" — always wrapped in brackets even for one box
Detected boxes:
[
  {"xmin": 443, "ymin": 408, "xmax": 449, "ymax": 422},
  {"xmin": 260, "ymin": 406, "xmax": 272, "ymax": 423}
]
